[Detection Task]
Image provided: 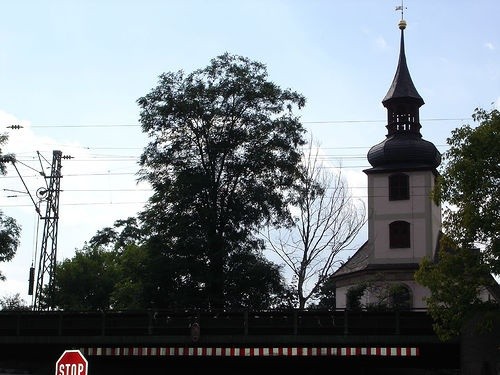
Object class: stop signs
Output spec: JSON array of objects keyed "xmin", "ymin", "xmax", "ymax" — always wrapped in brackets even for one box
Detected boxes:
[{"xmin": 55, "ymin": 349, "xmax": 89, "ymax": 375}]
